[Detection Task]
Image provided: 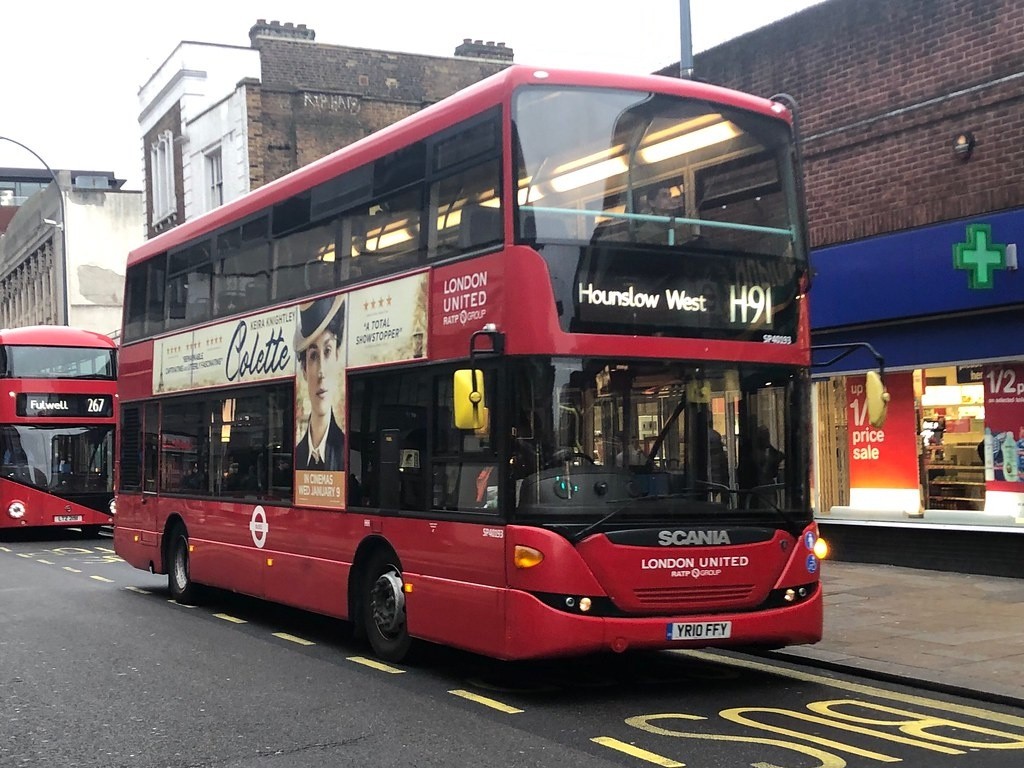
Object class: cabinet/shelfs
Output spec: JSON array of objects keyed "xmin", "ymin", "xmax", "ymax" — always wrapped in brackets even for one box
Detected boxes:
[{"xmin": 925, "ymin": 445, "xmax": 987, "ymax": 510}]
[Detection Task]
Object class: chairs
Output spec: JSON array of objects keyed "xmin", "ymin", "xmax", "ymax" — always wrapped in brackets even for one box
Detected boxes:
[{"xmin": 151, "ymin": 199, "xmax": 651, "ymax": 332}]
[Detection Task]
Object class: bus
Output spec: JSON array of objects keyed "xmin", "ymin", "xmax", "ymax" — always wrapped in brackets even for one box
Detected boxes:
[
  {"xmin": 1, "ymin": 325, "xmax": 120, "ymax": 541},
  {"xmin": 109, "ymin": 65, "xmax": 828, "ymax": 664}
]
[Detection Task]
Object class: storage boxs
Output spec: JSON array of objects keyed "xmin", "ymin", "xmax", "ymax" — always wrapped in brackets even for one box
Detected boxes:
[
  {"xmin": 961, "ymin": 416, "xmax": 976, "ymax": 419},
  {"xmin": 970, "ymin": 419, "xmax": 983, "ymax": 431},
  {"xmin": 945, "ymin": 418, "xmax": 970, "ymax": 433}
]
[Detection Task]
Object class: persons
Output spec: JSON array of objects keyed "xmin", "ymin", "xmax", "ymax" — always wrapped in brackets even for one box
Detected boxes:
[
  {"xmin": 290, "ymin": 300, "xmax": 345, "ymax": 473},
  {"xmin": 739, "ymin": 423, "xmax": 784, "ymax": 509},
  {"xmin": 706, "ymin": 423, "xmax": 728, "ymax": 503},
  {"xmin": 636, "ymin": 178, "xmax": 693, "ymax": 247},
  {"xmin": 616, "ymin": 435, "xmax": 648, "ymax": 465}
]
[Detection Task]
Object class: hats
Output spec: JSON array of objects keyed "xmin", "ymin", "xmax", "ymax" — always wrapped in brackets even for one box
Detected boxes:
[{"xmin": 292, "ymin": 292, "xmax": 345, "ymax": 352}]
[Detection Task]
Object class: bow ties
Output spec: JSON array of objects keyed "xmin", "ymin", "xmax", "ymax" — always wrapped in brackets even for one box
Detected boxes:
[{"xmin": 307, "ymin": 455, "xmax": 325, "ymax": 471}]
